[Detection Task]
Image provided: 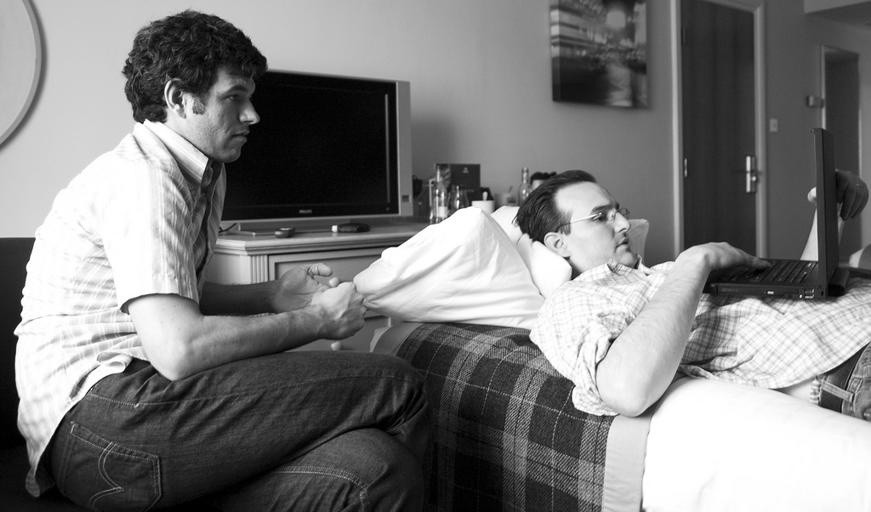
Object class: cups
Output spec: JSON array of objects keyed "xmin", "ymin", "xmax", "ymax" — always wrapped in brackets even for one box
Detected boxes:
[
  {"xmin": 495, "ymin": 191, "xmax": 514, "ymax": 211},
  {"xmin": 472, "ymin": 200, "xmax": 495, "ymax": 214}
]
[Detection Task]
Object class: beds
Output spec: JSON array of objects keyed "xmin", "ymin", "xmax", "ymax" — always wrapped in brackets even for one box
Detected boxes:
[{"xmin": 359, "ymin": 294, "xmax": 869, "ymax": 512}]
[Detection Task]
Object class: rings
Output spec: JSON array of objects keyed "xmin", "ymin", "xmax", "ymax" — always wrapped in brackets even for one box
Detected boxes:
[{"xmin": 855, "ymin": 183, "xmax": 865, "ymax": 189}]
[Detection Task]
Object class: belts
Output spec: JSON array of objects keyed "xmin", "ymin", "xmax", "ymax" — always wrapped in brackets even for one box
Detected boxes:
[{"xmin": 818, "ymin": 339, "xmax": 871, "ymax": 414}]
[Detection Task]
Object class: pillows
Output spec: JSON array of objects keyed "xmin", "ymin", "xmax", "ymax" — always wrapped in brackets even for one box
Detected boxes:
[
  {"xmin": 489, "ymin": 201, "xmax": 649, "ymax": 296},
  {"xmin": 350, "ymin": 205, "xmax": 542, "ymax": 330}
]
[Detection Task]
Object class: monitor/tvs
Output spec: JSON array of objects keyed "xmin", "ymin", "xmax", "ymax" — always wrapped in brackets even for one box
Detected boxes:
[{"xmin": 219, "ymin": 67, "xmax": 412, "ymax": 236}]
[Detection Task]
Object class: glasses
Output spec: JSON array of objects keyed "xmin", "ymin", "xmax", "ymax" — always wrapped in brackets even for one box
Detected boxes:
[{"xmin": 554, "ymin": 205, "xmax": 630, "ymax": 231}]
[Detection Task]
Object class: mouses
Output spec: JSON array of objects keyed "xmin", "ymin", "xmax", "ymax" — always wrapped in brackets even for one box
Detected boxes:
[{"xmin": 274, "ymin": 224, "xmax": 295, "ymax": 239}]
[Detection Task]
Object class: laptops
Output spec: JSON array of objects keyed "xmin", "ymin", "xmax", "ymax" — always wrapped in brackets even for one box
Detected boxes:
[{"xmin": 702, "ymin": 128, "xmax": 850, "ymax": 301}]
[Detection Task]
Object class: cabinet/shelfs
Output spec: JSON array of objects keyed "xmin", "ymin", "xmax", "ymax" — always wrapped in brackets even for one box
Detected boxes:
[{"xmin": 199, "ymin": 222, "xmax": 429, "ymax": 286}]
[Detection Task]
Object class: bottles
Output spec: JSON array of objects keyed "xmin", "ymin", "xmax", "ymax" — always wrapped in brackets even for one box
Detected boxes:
[
  {"xmin": 432, "ymin": 164, "xmax": 449, "ymax": 223},
  {"xmin": 518, "ymin": 169, "xmax": 533, "ymax": 208},
  {"xmin": 451, "ymin": 184, "xmax": 469, "ymax": 212}
]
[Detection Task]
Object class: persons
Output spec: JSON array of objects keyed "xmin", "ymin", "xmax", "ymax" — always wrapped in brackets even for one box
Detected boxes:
[
  {"xmin": 12, "ymin": 8, "xmax": 444, "ymax": 511},
  {"xmin": 516, "ymin": 168, "xmax": 871, "ymax": 431}
]
